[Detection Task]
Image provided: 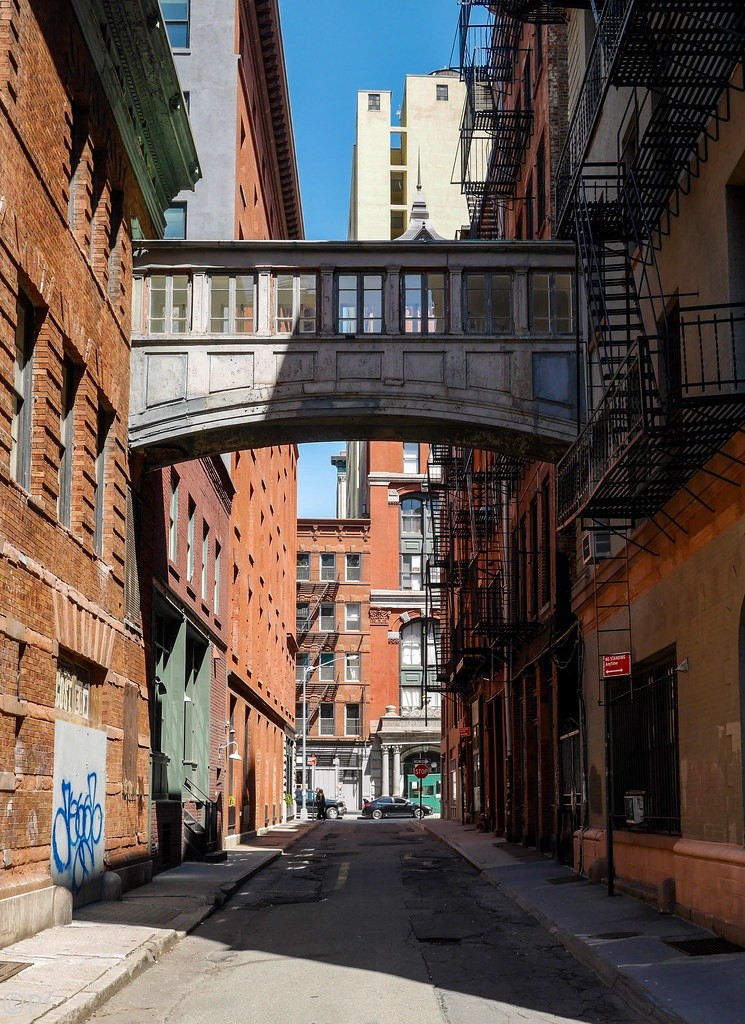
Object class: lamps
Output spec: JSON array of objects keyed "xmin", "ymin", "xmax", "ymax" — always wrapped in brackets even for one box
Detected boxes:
[{"xmin": 218, "ymin": 741, "xmax": 242, "ymax": 761}]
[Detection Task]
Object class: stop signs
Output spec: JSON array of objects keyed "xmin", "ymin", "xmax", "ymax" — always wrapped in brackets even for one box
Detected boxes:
[{"xmin": 415, "ymin": 764, "xmax": 429, "ymax": 778}]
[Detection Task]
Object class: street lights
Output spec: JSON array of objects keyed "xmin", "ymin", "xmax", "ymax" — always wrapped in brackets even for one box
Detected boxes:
[{"xmin": 300, "ymin": 654, "xmax": 360, "ymax": 818}]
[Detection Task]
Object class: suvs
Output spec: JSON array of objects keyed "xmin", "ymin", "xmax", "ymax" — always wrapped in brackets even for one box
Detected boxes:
[{"xmin": 296, "ymin": 789, "xmax": 347, "ymax": 819}]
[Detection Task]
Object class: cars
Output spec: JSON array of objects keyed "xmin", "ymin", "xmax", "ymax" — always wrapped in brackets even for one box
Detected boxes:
[{"xmin": 362, "ymin": 796, "xmax": 433, "ymax": 820}]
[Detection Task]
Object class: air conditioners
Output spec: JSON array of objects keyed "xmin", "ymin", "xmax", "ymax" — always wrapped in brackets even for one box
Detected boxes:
[{"xmin": 581, "ymin": 531, "xmax": 610, "ymax": 565}]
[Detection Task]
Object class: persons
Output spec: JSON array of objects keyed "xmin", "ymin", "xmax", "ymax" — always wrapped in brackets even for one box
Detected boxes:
[
  {"xmin": 315, "ymin": 788, "xmax": 326, "ymax": 820},
  {"xmin": 315, "ymin": 786, "xmax": 320, "ymax": 803}
]
